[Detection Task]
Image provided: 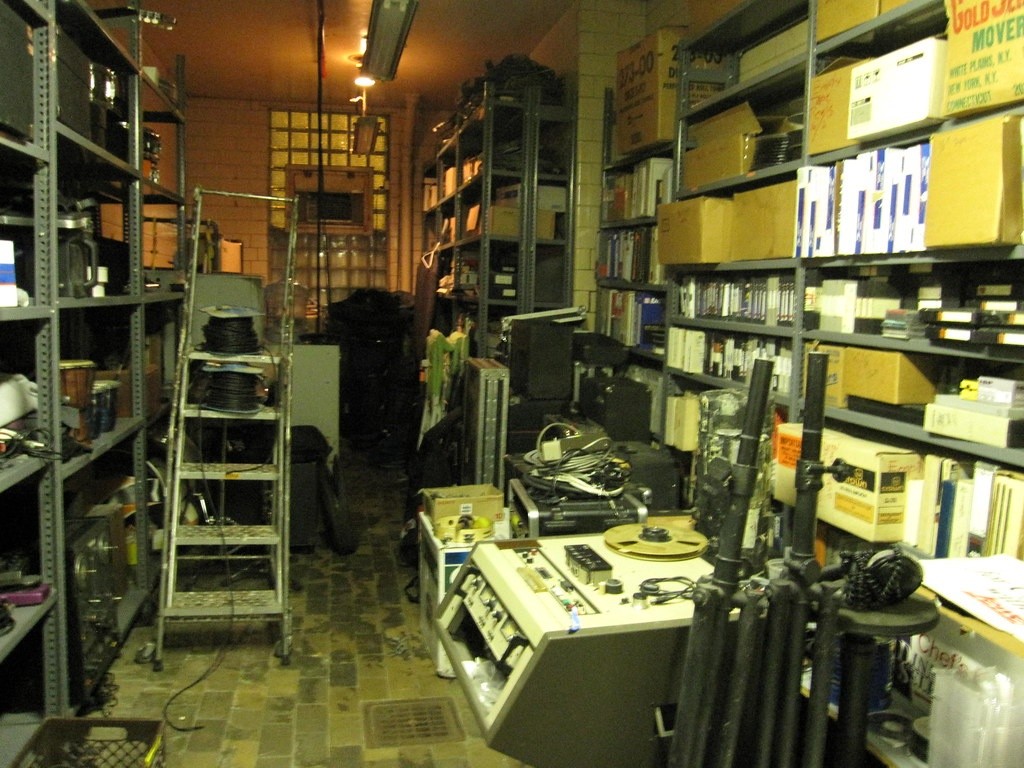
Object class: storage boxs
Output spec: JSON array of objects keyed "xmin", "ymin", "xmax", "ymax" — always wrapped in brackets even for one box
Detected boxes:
[
  {"xmin": 481, "ymin": 207, "xmax": 555, "ymax": 242},
  {"xmin": 806, "ymin": 59, "xmax": 877, "ymax": 158},
  {"xmin": 684, "ymin": 132, "xmax": 758, "ymax": 188},
  {"xmin": 941, "ymin": 0, "xmax": 1024, "ymax": 115},
  {"xmin": 803, "ymin": 343, "xmax": 846, "ymax": 407},
  {"xmin": 423, "ymin": 483, "xmax": 503, "ymax": 539},
  {"xmin": 843, "ymin": 346, "xmax": 936, "ymax": 405},
  {"xmin": 817, "ymin": 428, "xmax": 924, "ymax": 541},
  {"xmin": 95, "ymin": 364, "xmax": 161, "ymax": 418},
  {"xmin": 11, "ymin": 716, "xmax": 167, "ymax": 768},
  {"xmin": 657, "ymin": 198, "xmax": 730, "ymax": 262},
  {"xmin": 614, "ymin": 25, "xmax": 680, "ymax": 155},
  {"xmin": 215, "ymin": 234, "xmax": 246, "ymax": 273},
  {"xmin": 466, "ymin": 204, "xmax": 482, "ymax": 237},
  {"xmin": 815, "ymin": 0, "xmax": 879, "ymax": 44},
  {"xmin": 848, "ymin": 37, "xmax": 947, "ymax": 142},
  {"xmin": 924, "ymin": 113, "xmax": 1024, "ymax": 247},
  {"xmin": 731, "ymin": 179, "xmax": 796, "ymax": 263}
]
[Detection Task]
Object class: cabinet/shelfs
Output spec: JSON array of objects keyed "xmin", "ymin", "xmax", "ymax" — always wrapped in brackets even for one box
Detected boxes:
[
  {"xmin": 588, "ymin": 0, "xmax": 1024, "ymax": 768},
  {"xmin": 422, "ymin": 104, "xmax": 574, "ymax": 359},
  {"xmin": 0, "ymin": 0, "xmax": 188, "ymax": 768}
]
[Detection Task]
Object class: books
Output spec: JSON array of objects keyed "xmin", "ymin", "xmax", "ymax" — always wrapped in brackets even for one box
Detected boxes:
[
  {"xmin": 901, "ymin": 450, "xmax": 1024, "ymax": 566},
  {"xmin": 673, "ymin": 272, "xmax": 795, "ymax": 328},
  {"xmin": 604, "ymin": 224, "xmax": 669, "ymax": 286},
  {"xmin": 802, "ymin": 261, "xmax": 1024, "ymax": 348},
  {"xmin": 665, "ymin": 324, "xmax": 794, "ymax": 396},
  {"xmin": 923, "ymin": 373, "xmax": 1024, "ymax": 448},
  {"xmin": 791, "ymin": 145, "xmax": 934, "ymax": 258},
  {"xmin": 598, "ymin": 288, "xmax": 668, "ymax": 354},
  {"xmin": 604, "ymin": 155, "xmax": 673, "ymax": 220}
]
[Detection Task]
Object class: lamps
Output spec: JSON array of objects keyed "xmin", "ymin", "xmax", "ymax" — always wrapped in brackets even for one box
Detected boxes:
[
  {"xmin": 350, "ymin": 89, "xmax": 381, "ymax": 156},
  {"xmin": 360, "ymin": 0, "xmax": 416, "ymax": 81}
]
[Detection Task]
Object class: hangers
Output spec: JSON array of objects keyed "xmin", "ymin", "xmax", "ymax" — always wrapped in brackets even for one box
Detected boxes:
[{"xmin": 422, "ymin": 240, "xmax": 440, "ymax": 268}]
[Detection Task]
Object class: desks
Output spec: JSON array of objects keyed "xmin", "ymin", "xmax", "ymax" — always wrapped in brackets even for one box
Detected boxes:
[{"xmin": 823, "ymin": 596, "xmax": 939, "ymax": 768}]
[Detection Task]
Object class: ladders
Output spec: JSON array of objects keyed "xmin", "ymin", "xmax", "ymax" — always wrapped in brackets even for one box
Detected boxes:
[{"xmin": 153, "ymin": 185, "xmax": 301, "ymax": 672}]
